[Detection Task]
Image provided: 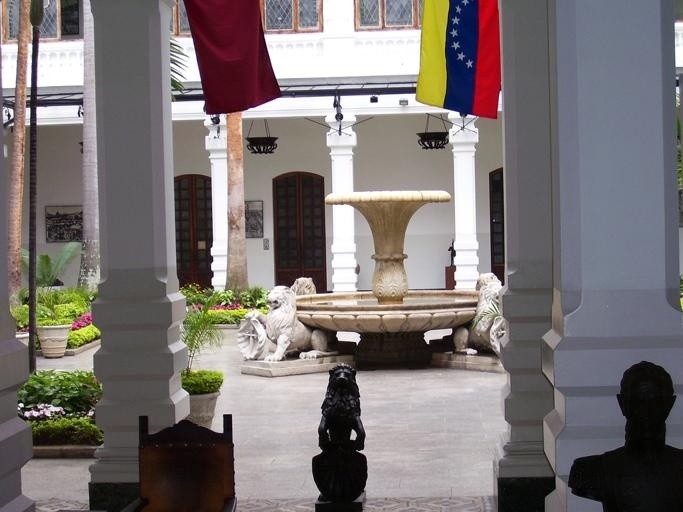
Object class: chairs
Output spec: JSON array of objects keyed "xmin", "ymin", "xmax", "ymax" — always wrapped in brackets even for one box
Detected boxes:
[{"xmin": 116, "ymin": 413, "xmax": 239, "ymax": 512}]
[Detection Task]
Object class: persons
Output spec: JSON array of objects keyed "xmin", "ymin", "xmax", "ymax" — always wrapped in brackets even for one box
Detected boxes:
[{"xmin": 562, "ymin": 357, "xmax": 683, "ymax": 512}]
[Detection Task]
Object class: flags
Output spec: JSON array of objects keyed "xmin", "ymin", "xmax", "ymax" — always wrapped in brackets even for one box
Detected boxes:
[
  {"xmin": 182, "ymin": 0, "xmax": 283, "ymax": 115},
  {"xmin": 414, "ymin": 0, "xmax": 503, "ymax": 120}
]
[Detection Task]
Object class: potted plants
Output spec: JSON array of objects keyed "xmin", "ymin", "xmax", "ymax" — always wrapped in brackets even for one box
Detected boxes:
[
  {"xmin": 180, "ymin": 369, "xmax": 226, "ymax": 428},
  {"xmin": 31, "ymin": 289, "xmax": 88, "ymax": 359}
]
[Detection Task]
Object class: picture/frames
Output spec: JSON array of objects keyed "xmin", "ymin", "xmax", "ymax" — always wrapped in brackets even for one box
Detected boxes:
[{"xmin": 45, "ymin": 203, "xmax": 85, "ymax": 243}]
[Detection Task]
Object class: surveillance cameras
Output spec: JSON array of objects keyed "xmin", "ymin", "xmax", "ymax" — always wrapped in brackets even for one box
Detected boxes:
[{"xmin": 399, "ymin": 97, "xmax": 408, "ymax": 105}]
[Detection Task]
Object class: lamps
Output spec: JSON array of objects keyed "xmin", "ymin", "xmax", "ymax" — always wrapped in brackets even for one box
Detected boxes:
[
  {"xmin": 416, "ymin": 116, "xmax": 450, "ymax": 150},
  {"xmin": 245, "ymin": 120, "xmax": 279, "ymax": 155}
]
